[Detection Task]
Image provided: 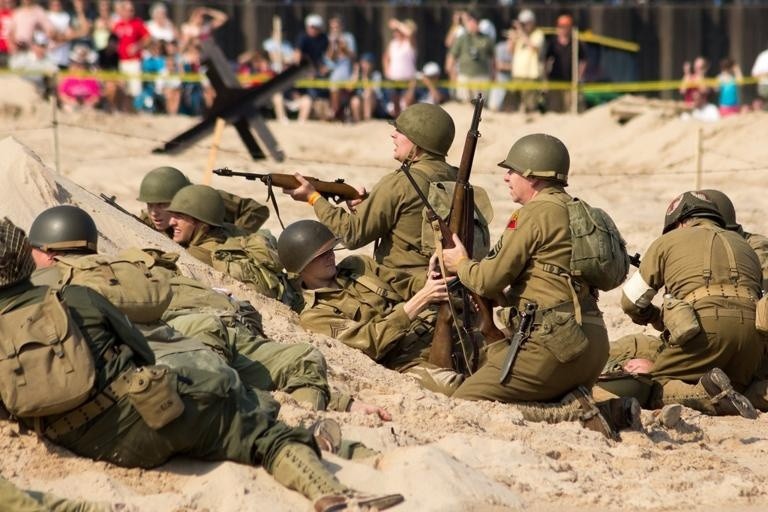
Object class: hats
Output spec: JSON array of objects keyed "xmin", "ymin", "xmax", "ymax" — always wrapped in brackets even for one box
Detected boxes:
[
  {"xmin": 69, "ymin": 44, "xmax": 99, "ymax": 64},
  {"xmin": 556, "ymin": 15, "xmax": 571, "ymax": 28},
  {"xmin": 305, "ymin": 15, "xmax": 325, "ymax": 28},
  {"xmin": 518, "ymin": 10, "xmax": 536, "ymax": 23}
]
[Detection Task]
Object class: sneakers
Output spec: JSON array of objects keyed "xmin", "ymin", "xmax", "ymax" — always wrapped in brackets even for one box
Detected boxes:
[
  {"xmin": 702, "ymin": 368, "xmax": 758, "ymax": 419},
  {"xmin": 572, "ymin": 386, "xmax": 611, "ymax": 439},
  {"xmin": 595, "ymin": 398, "xmax": 642, "ymax": 430},
  {"xmin": 313, "ymin": 489, "xmax": 403, "ymax": 512}
]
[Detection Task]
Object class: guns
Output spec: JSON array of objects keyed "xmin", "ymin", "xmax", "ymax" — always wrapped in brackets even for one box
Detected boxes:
[
  {"xmin": 100, "ymin": 193, "xmax": 160, "ymax": 231},
  {"xmin": 401, "ymin": 165, "xmax": 511, "ymax": 345},
  {"xmin": 427, "ymin": 92, "xmax": 484, "ymax": 372},
  {"xmin": 213, "ymin": 167, "xmax": 365, "ymax": 204}
]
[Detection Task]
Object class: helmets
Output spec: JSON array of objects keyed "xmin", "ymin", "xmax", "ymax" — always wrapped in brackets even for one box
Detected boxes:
[
  {"xmin": 278, "ymin": 220, "xmax": 336, "ymax": 274},
  {"xmin": 597, "ymin": 372, "xmax": 652, "ymax": 409},
  {"xmin": 0, "ymin": 217, "xmax": 36, "ymax": 285},
  {"xmin": 28, "ymin": 206, "xmax": 98, "ymax": 255},
  {"xmin": 497, "ymin": 133, "xmax": 570, "ymax": 188},
  {"xmin": 136, "ymin": 167, "xmax": 188, "ymax": 203},
  {"xmin": 387, "ymin": 103, "xmax": 455, "ymax": 156},
  {"xmin": 700, "ymin": 190, "xmax": 740, "ymax": 229},
  {"xmin": 162, "ymin": 184, "xmax": 227, "ymax": 228},
  {"xmin": 662, "ymin": 190, "xmax": 726, "ymax": 234}
]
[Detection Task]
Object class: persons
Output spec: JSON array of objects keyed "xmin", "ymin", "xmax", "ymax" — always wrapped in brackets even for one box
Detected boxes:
[
  {"xmin": 1, "ymin": 206, "xmax": 404, "ymax": 511},
  {"xmin": 681, "ymin": 49, "xmax": 768, "ymax": 117},
  {"xmin": 1, "ymin": 1, "xmax": 587, "ymax": 125},
  {"xmin": 139, "ymin": 103, "xmax": 768, "ymax": 441}
]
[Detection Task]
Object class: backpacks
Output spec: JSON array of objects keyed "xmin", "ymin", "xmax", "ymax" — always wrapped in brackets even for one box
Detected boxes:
[
  {"xmin": 213, "ymin": 230, "xmax": 278, "ymax": 298},
  {"xmin": 531, "ymin": 196, "xmax": 631, "ymax": 292},
  {"xmin": 1, "ymin": 286, "xmax": 96, "ymax": 418},
  {"xmin": 50, "ymin": 247, "xmax": 173, "ymax": 325},
  {"xmin": 407, "ymin": 161, "xmax": 494, "ymax": 264}
]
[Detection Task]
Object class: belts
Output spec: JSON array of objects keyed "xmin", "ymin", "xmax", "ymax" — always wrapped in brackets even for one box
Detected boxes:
[
  {"xmin": 47, "ymin": 365, "xmax": 137, "ymax": 437},
  {"xmin": 697, "ymin": 309, "xmax": 755, "ymax": 318}
]
